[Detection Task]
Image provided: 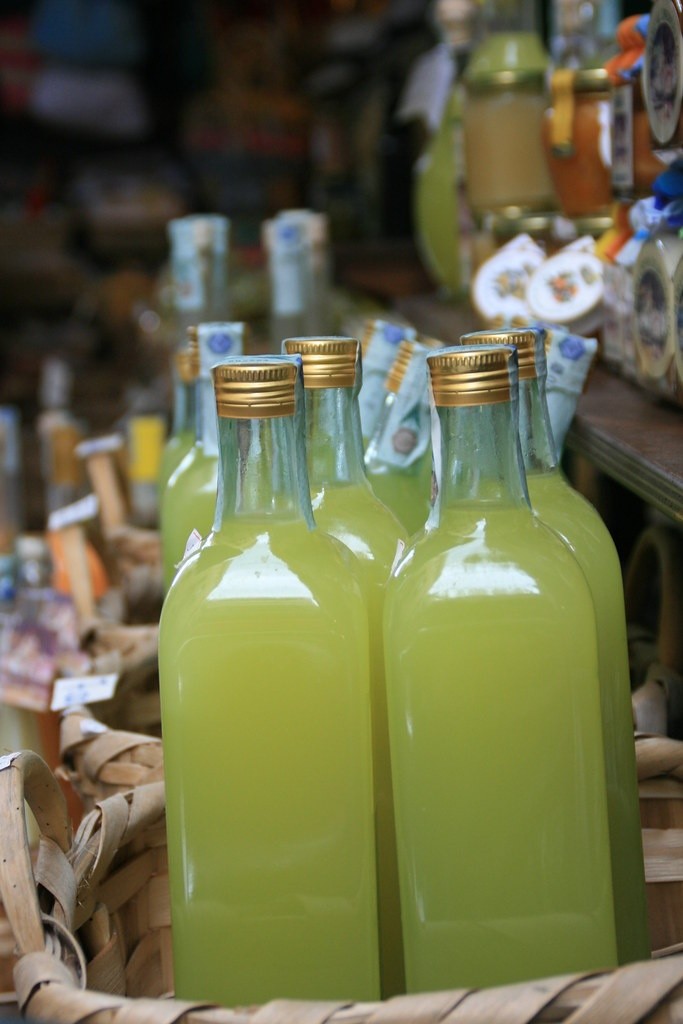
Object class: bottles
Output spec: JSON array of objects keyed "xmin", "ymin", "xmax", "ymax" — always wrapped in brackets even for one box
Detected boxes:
[{"xmin": 154, "ymin": 0, "xmax": 683, "ymax": 1001}]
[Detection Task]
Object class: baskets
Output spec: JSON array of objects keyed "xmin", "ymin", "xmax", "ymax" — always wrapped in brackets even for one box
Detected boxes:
[
  {"xmin": 91, "ymin": 439, "xmax": 166, "ymax": 562},
  {"xmin": 59, "ymin": 521, "xmax": 170, "ymax": 651},
  {"xmin": 53, "ymin": 526, "xmax": 679, "ymax": 796},
  {"xmin": 0, "ymin": 732, "xmax": 683, "ymax": 1024}
]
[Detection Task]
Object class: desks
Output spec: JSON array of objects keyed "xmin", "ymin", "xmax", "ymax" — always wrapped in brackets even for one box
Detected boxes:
[{"xmin": 400, "ymin": 292, "xmax": 683, "ymax": 664}]
